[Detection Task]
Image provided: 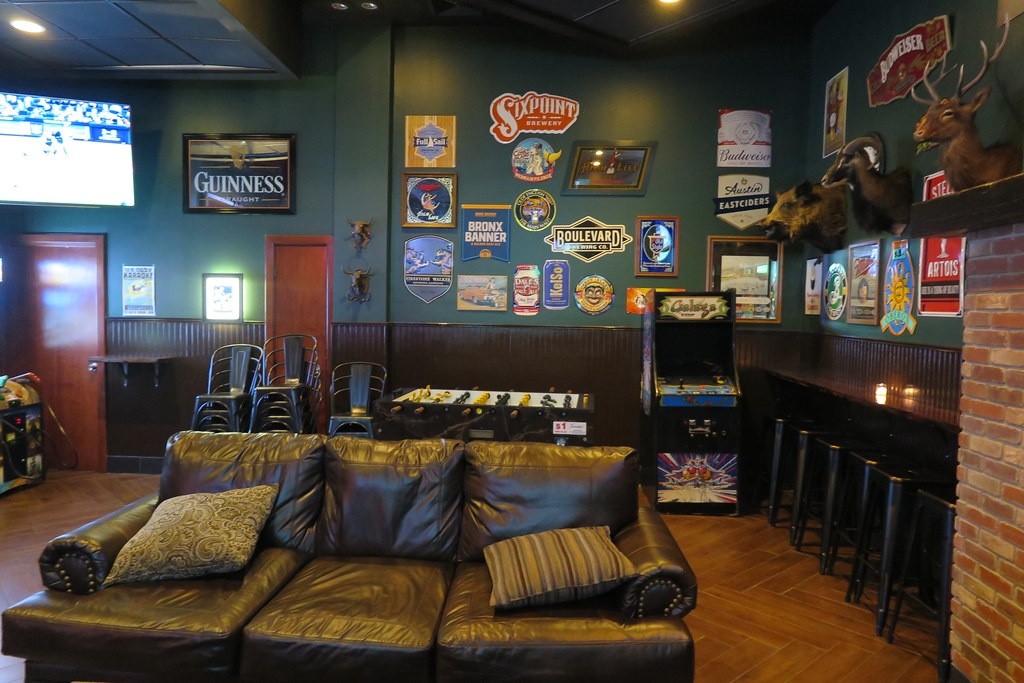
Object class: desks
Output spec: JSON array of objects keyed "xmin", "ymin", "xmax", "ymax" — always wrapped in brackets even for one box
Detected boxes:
[{"xmin": 89, "ymin": 353, "xmax": 182, "ymax": 388}]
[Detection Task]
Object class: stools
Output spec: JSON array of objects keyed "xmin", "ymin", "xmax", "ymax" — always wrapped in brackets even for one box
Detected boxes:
[{"xmin": 763, "ymin": 411, "xmax": 957, "ymax": 683}]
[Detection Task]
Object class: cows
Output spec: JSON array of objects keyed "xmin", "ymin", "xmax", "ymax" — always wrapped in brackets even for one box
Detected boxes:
[
  {"xmin": 347, "ymin": 216, "xmax": 375, "ymax": 244},
  {"xmin": 342, "ymin": 265, "xmax": 372, "ymax": 294}
]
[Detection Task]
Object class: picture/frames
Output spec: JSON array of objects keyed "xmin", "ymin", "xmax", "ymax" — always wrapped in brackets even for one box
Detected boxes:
[
  {"xmin": 706, "ymin": 235, "xmax": 784, "ymax": 324},
  {"xmin": 845, "ymin": 238, "xmax": 882, "ymax": 327},
  {"xmin": 634, "ymin": 215, "xmax": 680, "ymax": 278},
  {"xmin": 202, "ymin": 272, "xmax": 243, "ymax": 325},
  {"xmin": 182, "ymin": 131, "xmax": 298, "ymax": 216},
  {"xmin": 559, "ymin": 140, "xmax": 658, "ymax": 196},
  {"xmin": 401, "ymin": 171, "xmax": 458, "ymax": 229}
]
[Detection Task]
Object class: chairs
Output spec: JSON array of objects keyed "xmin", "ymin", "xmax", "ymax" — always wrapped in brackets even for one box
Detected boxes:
[{"xmin": 193, "ymin": 333, "xmax": 388, "ymax": 441}]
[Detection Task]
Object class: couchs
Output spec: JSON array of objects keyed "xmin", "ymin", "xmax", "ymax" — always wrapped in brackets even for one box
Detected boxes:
[{"xmin": 0, "ymin": 429, "xmax": 698, "ymax": 683}]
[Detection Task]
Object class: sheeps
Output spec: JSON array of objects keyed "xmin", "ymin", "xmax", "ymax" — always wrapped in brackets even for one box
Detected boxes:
[{"xmin": 821, "ymin": 132, "xmax": 913, "ymax": 233}]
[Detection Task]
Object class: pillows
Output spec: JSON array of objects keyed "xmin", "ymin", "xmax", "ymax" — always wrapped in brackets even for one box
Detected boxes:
[
  {"xmin": 482, "ymin": 522, "xmax": 640, "ymax": 607},
  {"xmin": 102, "ymin": 480, "xmax": 279, "ymax": 590}
]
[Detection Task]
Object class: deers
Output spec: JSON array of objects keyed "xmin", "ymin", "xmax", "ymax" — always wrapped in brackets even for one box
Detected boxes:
[{"xmin": 911, "ymin": 11, "xmax": 1024, "ymax": 192}]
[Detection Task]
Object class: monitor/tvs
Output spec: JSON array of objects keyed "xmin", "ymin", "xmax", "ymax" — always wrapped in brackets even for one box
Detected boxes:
[{"xmin": 0, "ymin": 91, "xmax": 134, "ymax": 208}]
